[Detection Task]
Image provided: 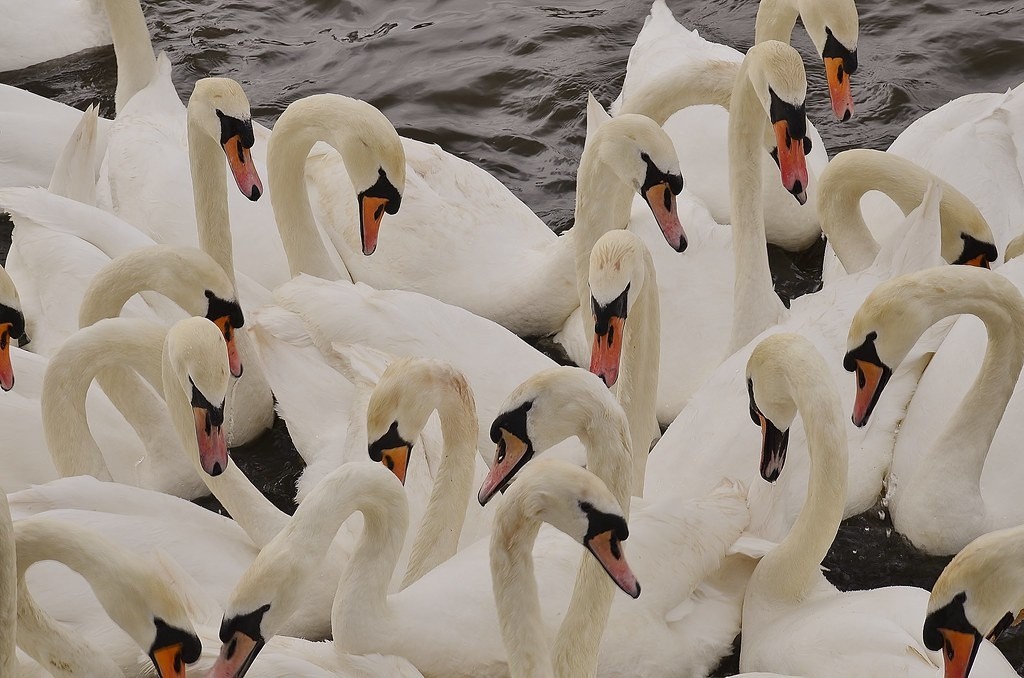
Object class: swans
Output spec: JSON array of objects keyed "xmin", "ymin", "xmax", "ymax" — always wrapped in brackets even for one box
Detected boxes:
[{"xmin": 0, "ymin": 0, "xmax": 1023, "ymax": 678}]
